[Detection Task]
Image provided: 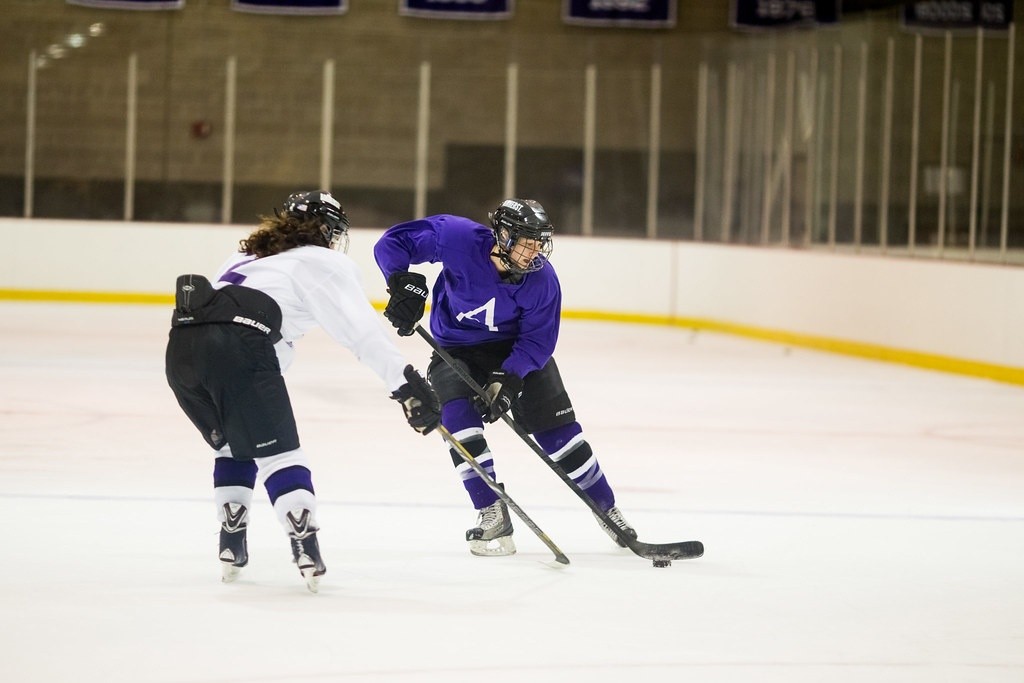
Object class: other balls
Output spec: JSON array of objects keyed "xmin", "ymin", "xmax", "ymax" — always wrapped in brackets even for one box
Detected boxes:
[{"xmin": 652, "ymin": 559, "xmax": 672, "ymax": 567}]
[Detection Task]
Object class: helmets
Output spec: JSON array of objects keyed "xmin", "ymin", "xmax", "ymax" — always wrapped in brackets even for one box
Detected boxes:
[
  {"xmin": 283, "ymin": 190, "xmax": 349, "ymax": 257},
  {"xmin": 489, "ymin": 200, "xmax": 555, "ymax": 275}
]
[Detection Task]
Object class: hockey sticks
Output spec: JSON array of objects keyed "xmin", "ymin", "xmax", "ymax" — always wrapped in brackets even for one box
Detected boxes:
[
  {"xmin": 414, "ymin": 321, "xmax": 706, "ymax": 563},
  {"xmin": 410, "ymin": 395, "xmax": 573, "ymax": 567}
]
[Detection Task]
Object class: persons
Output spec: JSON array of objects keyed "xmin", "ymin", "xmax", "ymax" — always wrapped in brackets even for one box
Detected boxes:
[
  {"xmin": 374, "ymin": 198, "xmax": 639, "ymax": 554},
  {"xmin": 166, "ymin": 188, "xmax": 442, "ymax": 595}
]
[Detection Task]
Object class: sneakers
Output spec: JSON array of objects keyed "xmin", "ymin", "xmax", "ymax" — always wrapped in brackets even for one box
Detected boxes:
[
  {"xmin": 465, "ymin": 498, "xmax": 516, "ymax": 556},
  {"xmin": 217, "ymin": 520, "xmax": 249, "ymax": 583},
  {"xmin": 593, "ymin": 506, "xmax": 638, "ymax": 548},
  {"xmin": 289, "ymin": 526, "xmax": 326, "ymax": 594}
]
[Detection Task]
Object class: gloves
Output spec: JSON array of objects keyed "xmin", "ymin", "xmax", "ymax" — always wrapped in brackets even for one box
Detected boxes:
[
  {"xmin": 472, "ymin": 368, "xmax": 525, "ymax": 423},
  {"xmin": 390, "ymin": 365, "xmax": 442, "ymax": 436},
  {"xmin": 383, "ymin": 271, "xmax": 429, "ymax": 336}
]
